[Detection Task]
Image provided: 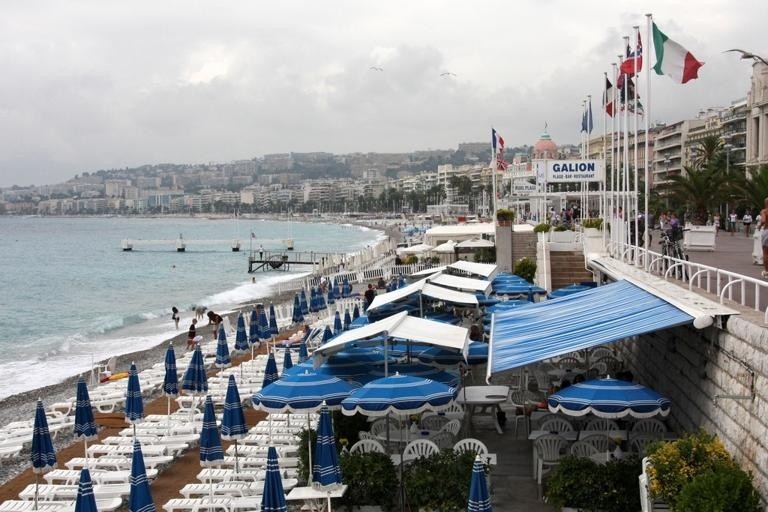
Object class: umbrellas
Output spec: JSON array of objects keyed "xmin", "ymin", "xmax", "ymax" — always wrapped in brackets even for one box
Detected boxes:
[
  {"xmin": 550, "ymin": 374, "xmax": 672, "ymax": 464},
  {"xmin": 547, "ymin": 281, "xmax": 592, "ymax": 299}
]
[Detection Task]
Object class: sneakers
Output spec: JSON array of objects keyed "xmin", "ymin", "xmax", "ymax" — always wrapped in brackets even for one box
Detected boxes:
[{"xmin": 761, "ymin": 271, "xmax": 768, "ymax": 278}]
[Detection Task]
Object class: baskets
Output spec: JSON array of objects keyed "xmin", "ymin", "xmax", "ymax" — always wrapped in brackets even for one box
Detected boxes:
[{"xmin": 666, "ymin": 227, "xmax": 683, "ymax": 242}]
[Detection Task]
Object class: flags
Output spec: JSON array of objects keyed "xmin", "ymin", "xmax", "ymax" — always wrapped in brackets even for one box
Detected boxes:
[
  {"xmin": 602, "ymin": 32, "xmax": 645, "ymax": 117},
  {"xmin": 489, "ymin": 130, "xmax": 510, "ymax": 173},
  {"xmin": 579, "ymin": 98, "xmax": 594, "ymax": 132},
  {"xmin": 650, "ymin": 21, "xmax": 706, "ymax": 84}
]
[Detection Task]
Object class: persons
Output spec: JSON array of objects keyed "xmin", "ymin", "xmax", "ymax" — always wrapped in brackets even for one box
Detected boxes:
[
  {"xmin": 258, "ymin": 245, "xmax": 265, "ymax": 259},
  {"xmin": 707, "ymin": 198, "xmax": 768, "ymax": 279},
  {"xmin": 659, "ymin": 209, "xmax": 679, "ymax": 235},
  {"xmin": 252, "ymin": 277, "xmax": 256, "ymax": 283},
  {"xmin": 364, "ymin": 276, "xmax": 392, "ymax": 305},
  {"xmin": 606, "ymin": 202, "xmax": 654, "ymax": 246},
  {"xmin": 548, "ymin": 202, "xmax": 582, "ymax": 222},
  {"xmin": 171, "ymin": 304, "xmax": 224, "ymax": 350}
]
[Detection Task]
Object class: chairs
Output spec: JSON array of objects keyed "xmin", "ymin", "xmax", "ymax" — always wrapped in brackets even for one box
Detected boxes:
[
  {"xmin": 510, "ymin": 347, "xmax": 674, "ymax": 512},
  {"xmin": 350, "ymin": 400, "xmax": 497, "ymax": 494},
  {"xmin": 0, "ymin": 312, "xmax": 363, "ymax": 512}
]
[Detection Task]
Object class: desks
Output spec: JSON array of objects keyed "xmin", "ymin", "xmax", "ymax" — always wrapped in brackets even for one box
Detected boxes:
[
  {"xmin": 286, "ymin": 485, "xmax": 348, "ymax": 512},
  {"xmin": 456, "ymin": 386, "xmax": 510, "ymax": 434}
]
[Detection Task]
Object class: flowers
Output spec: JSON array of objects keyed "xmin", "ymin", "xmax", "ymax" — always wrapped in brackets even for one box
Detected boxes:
[{"xmin": 645, "ymin": 427, "xmax": 733, "ymax": 500}]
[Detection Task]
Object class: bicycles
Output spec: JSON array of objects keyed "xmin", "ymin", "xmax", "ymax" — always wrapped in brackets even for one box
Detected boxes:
[
  {"xmin": 659, "ymin": 226, "xmax": 690, "ymax": 280},
  {"xmin": 629, "ymin": 220, "xmax": 652, "ymax": 262}
]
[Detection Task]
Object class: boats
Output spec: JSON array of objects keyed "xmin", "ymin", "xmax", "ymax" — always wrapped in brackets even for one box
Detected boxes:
[
  {"xmin": 98, "ymin": 372, "xmax": 129, "ymax": 383},
  {"xmin": 122, "ymin": 235, "xmax": 240, "ymax": 251}
]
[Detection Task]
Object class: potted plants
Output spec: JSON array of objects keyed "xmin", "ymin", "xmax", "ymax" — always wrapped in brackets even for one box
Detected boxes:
[
  {"xmin": 581, "ymin": 218, "xmax": 603, "ymax": 237},
  {"xmin": 655, "ymin": 149, "xmax": 768, "ymax": 265},
  {"xmin": 534, "ymin": 223, "xmax": 552, "ymax": 241},
  {"xmin": 496, "ymin": 209, "xmax": 517, "ymax": 226},
  {"xmin": 553, "ymin": 221, "xmax": 575, "ymax": 243}
]
